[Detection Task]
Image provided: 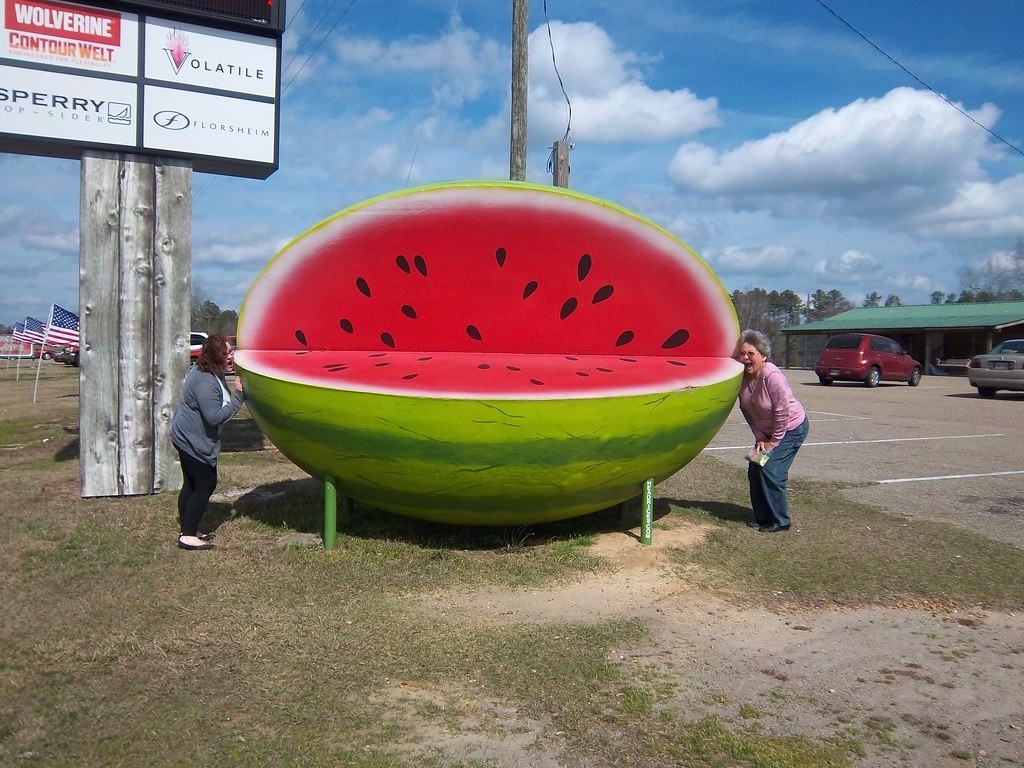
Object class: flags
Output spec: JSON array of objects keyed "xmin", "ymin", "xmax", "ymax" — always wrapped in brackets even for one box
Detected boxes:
[
  {"xmin": 44, "ymin": 304, "xmax": 80, "ymax": 348},
  {"xmin": 22, "ymin": 317, "xmax": 46, "ymax": 344},
  {"xmin": 12, "ymin": 322, "xmax": 24, "ymax": 341}
]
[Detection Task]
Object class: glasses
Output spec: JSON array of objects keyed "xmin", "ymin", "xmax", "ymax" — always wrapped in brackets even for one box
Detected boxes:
[
  {"xmin": 224, "ymin": 348, "xmax": 235, "ymax": 358},
  {"xmin": 737, "ymin": 350, "xmax": 754, "ymax": 356}
]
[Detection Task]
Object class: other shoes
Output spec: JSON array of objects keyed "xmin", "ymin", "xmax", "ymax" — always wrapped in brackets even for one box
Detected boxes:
[
  {"xmin": 177, "ymin": 533, "xmax": 214, "ymax": 550},
  {"xmin": 196, "ymin": 529, "xmax": 217, "ymax": 541},
  {"xmin": 759, "ymin": 522, "xmax": 792, "ymax": 532},
  {"xmin": 749, "ymin": 519, "xmax": 762, "ymax": 526}
]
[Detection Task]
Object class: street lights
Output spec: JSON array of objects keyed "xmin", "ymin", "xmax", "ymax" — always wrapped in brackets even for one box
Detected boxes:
[{"xmin": 204, "ymin": 314, "xmax": 214, "ymax": 335}]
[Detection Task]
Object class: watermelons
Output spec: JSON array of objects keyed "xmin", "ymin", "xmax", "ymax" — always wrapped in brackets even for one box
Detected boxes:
[{"xmin": 232, "ymin": 178, "xmax": 744, "ymax": 530}]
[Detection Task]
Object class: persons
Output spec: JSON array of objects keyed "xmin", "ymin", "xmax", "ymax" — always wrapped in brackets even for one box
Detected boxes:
[
  {"xmin": 170, "ymin": 333, "xmax": 244, "ymax": 549},
  {"xmin": 737, "ymin": 330, "xmax": 809, "ymax": 532}
]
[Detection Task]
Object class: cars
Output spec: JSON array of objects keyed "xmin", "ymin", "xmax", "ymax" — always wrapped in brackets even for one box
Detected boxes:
[
  {"xmin": 190, "ymin": 332, "xmax": 209, "ymax": 367},
  {"xmin": 31, "ymin": 347, "xmax": 80, "ymax": 366},
  {"xmin": 813, "ymin": 333, "xmax": 923, "ymax": 388},
  {"xmin": 967, "ymin": 339, "xmax": 1024, "ymax": 398}
]
